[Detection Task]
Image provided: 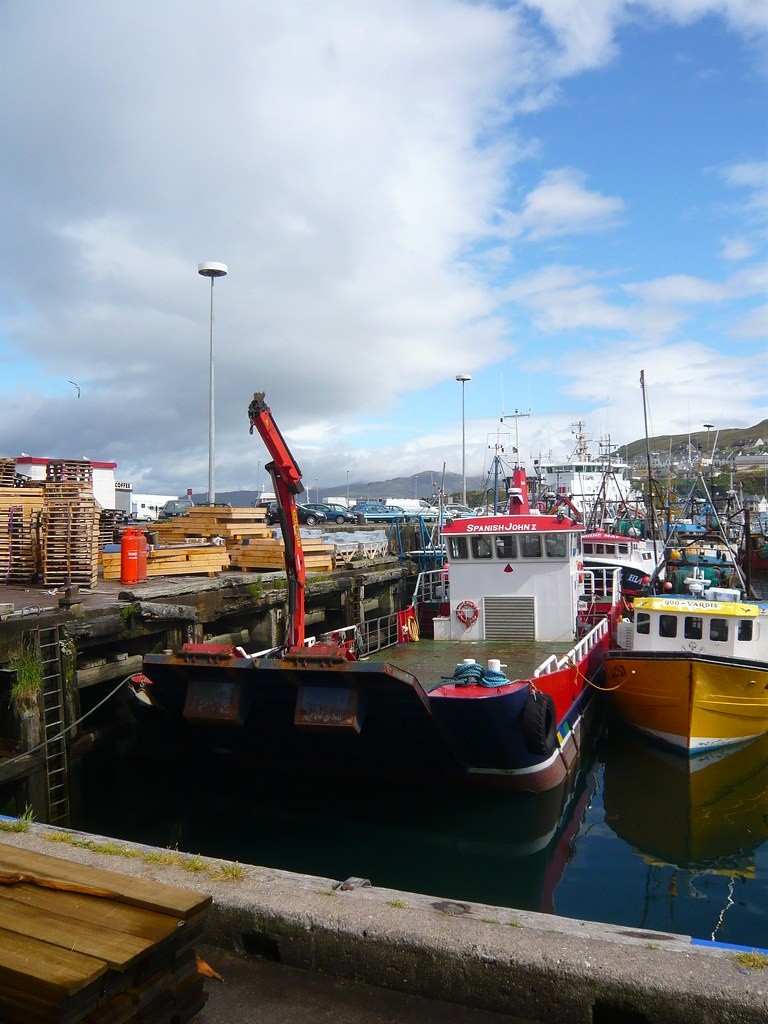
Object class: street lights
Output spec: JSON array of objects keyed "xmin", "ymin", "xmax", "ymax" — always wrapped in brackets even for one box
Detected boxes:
[
  {"xmin": 314, "ymin": 477, "xmax": 320, "ymax": 503},
  {"xmin": 413, "ymin": 474, "xmax": 419, "ymax": 498},
  {"xmin": 256, "ymin": 460, "xmax": 263, "ymax": 497},
  {"xmin": 455, "ymin": 374, "xmax": 473, "ymax": 508},
  {"xmin": 196, "ymin": 260, "xmax": 228, "ymax": 507},
  {"xmin": 346, "ymin": 469, "xmax": 350, "ymax": 506}
]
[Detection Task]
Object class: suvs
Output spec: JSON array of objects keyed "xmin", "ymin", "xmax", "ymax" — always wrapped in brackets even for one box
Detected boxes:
[{"xmin": 255, "ymin": 501, "xmax": 327, "ymax": 526}]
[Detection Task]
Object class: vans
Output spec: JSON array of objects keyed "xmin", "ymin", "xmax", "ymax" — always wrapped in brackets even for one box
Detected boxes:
[
  {"xmin": 381, "ymin": 498, "xmax": 440, "ymax": 518},
  {"xmin": 194, "ymin": 502, "xmax": 232, "ymax": 508}
]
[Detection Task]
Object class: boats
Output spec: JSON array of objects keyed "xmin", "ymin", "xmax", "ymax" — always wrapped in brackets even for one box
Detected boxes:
[
  {"xmin": 122, "ymin": 418, "xmax": 627, "ymax": 795},
  {"xmin": 564, "ymin": 419, "xmax": 768, "ymax": 755}
]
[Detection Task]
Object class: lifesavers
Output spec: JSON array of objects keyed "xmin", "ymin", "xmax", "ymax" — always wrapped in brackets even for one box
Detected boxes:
[
  {"xmin": 577, "ymin": 562, "xmax": 584, "ymax": 583},
  {"xmin": 455, "ymin": 601, "xmax": 478, "ymax": 624},
  {"xmin": 532, "ymin": 501, "xmax": 546, "ymax": 513}
]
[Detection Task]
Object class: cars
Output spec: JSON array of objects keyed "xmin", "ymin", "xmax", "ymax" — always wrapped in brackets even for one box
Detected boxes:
[
  {"xmin": 157, "ymin": 499, "xmax": 195, "ymax": 520},
  {"xmin": 351, "ymin": 505, "xmax": 414, "ymax": 525},
  {"xmin": 300, "ymin": 502, "xmax": 354, "ymax": 526},
  {"xmin": 324, "ymin": 503, "xmax": 359, "ymax": 524}
]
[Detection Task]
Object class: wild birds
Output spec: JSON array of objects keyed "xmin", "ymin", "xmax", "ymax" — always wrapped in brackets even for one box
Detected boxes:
[{"xmin": 69, "ymin": 380, "xmax": 80, "ymax": 400}]
